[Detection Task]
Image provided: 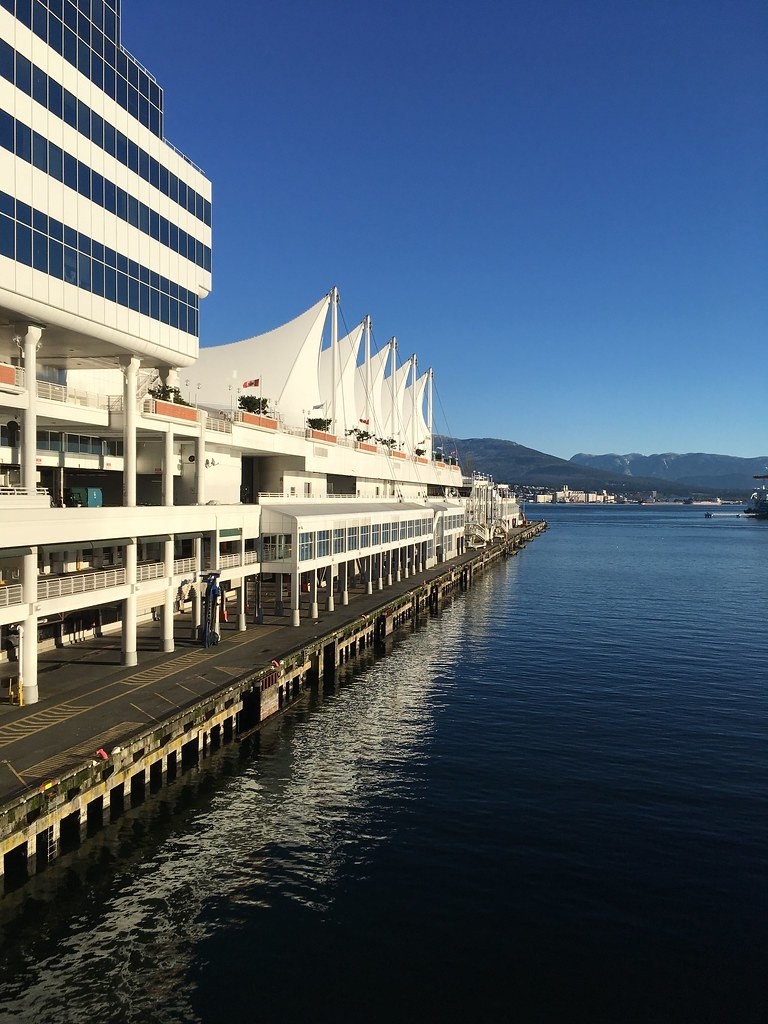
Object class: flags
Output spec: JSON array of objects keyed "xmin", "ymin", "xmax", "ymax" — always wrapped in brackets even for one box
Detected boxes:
[
  {"xmin": 312, "ymin": 403, "xmax": 326, "ymax": 409},
  {"xmin": 437, "ymin": 447, "xmax": 443, "ymax": 450},
  {"xmin": 360, "ymin": 419, "xmax": 369, "ymax": 424},
  {"xmin": 451, "ymin": 451, "xmax": 457, "ymax": 455},
  {"xmin": 243, "ymin": 379, "xmax": 259, "ymax": 388}
]
